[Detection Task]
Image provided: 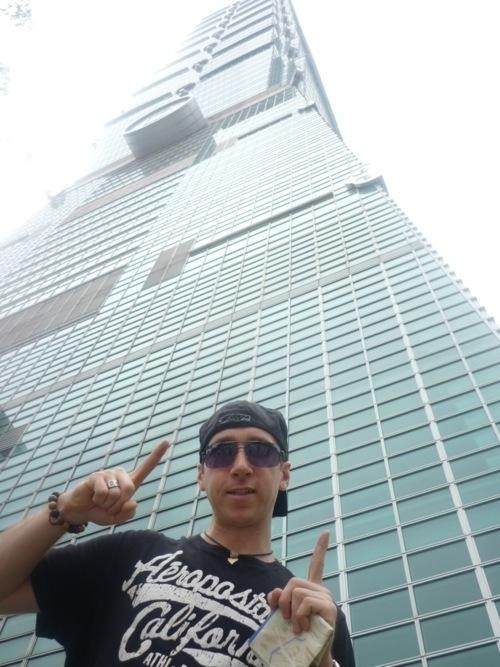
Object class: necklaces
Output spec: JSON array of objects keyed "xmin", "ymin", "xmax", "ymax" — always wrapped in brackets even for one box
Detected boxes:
[{"xmin": 203, "ymin": 528, "xmax": 273, "ymax": 565}]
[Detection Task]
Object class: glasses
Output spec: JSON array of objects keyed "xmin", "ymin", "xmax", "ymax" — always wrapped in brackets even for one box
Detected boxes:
[{"xmin": 198, "ymin": 440, "xmax": 288, "ymax": 467}]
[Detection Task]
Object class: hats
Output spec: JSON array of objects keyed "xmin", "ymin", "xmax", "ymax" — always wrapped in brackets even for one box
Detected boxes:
[{"xmin": 199, "ymin": 400, "xmax": 289, "ymax": 453}]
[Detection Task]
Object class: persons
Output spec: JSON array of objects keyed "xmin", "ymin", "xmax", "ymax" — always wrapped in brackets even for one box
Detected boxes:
[{"xmin": 0, "ymin": 399, "xmax": 355, "ymax": 667}]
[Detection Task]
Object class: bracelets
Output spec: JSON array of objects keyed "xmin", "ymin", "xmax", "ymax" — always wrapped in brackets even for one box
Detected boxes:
[
  {"xmin": 48, "ymin": 492, "xmax": 87, "ymax": 534},
  {"xmin": 332, "ymin": 658, "xmax": 340, "ymax": 667}
]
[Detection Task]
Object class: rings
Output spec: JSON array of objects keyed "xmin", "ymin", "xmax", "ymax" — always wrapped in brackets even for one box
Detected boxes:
[{"xmin": 106, "ymin": 479, "xmax": 120, "ymax": 489}]
